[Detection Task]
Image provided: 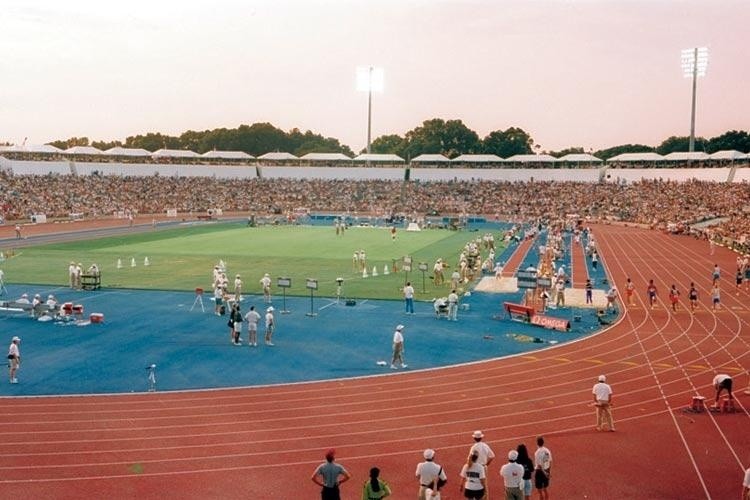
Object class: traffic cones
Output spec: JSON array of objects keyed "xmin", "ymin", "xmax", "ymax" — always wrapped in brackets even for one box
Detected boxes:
[
  {"xmin": 363, "ymin": 267, "xmax": 369, "ymax": 278},
  {"xmin": 383, "ymin": 264, "xmax": 389, "ymax": 275},
  {"xmin": 130, "ymin": 257, "xmax": 138, "ymax": 267},
  {"xmin": 143, "ymin": 256, "xmax": 151, "ymax": 266},
  {"xmin": 116, "ymin": 258, "xmax": 124, "ymax": 269},
  {"xmin": 372, "ymin": 266, "xmax": 378, "ymax": 277}
]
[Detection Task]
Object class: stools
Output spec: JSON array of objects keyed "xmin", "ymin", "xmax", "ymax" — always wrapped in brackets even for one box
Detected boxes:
[
  {"xmin": 691, "ymin": 395, "xmax": 706, "ymax": 411},
  {"xmin": 720, "ymin": 393, "xmax": 735, "ymax": 412}
]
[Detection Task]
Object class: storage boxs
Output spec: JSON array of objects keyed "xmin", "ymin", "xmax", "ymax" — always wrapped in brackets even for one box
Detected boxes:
[{"xmin": 90, "ymin": 312, "xmax": 104, "ymax": 323}]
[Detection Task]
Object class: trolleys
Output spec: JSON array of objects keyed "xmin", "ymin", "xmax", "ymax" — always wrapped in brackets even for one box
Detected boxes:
[{"xmin": 80, "ymin": 269, "xmax": 102, "ymax": 291}]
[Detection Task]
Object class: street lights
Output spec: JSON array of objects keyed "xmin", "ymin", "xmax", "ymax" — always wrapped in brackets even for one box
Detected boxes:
[
  {"xmin": 679, "ymin": 46, "xmax": 710, "ymax": 168},
  {"xmin": 354, "ymin": 64, "xmax": 385, "ymax": 168}
]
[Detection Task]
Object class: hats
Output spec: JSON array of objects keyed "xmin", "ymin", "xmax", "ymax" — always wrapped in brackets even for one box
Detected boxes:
[
  {"xmin": 325, "ymin": 447, "xmax": 336, "ymax": 460},
  {"xmin": 423, "ymin": 450, "xmax": 435, "ymax": 460},
  {"xmin": 598, "ymin": 375, "xmax": 606, "ymax": 382},
  {"xmin": 509, "ymin": 450, "xmax": 519, "ymax": 460},
  {"xmin": 473, "ymin": 430, "xmax": 485, "ymax": 439}
]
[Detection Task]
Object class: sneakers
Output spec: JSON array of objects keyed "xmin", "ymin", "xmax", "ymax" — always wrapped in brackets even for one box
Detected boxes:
[
  {"xmin": 232, "ymin": 337, "xmax": 276, "ymax": 347},
  {"xmin": 389, "ymin": 364, "xmax": 408, "ymax": 370}
]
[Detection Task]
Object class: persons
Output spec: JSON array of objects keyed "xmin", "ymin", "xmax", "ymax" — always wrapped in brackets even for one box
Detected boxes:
[
  {"xmin": 467, "ymin": 430, "xmax": 495, "ymax": 500},
  {"xmin": 516, "ymin": 444, "xmax": 534, "ymax": 500},
  {"xmin": 8, "ymin": 336, "xmax": 21, "ymax": 383},
  {"xmin": 534, "ymin": 436, "xmax": 553, "ymax": 500},
  {"xmin": 742, "ymin": 468, "xmax": 750, "ymax": 500},
  {"xmin": 592, "ymin": 375, "xmax": 616, "ymax": 431},
  {"xmin": 416, "ymin": 448, "xmax": 447, "ymax": 500},
  {"xmin": 390, "ymin": 325, "xmax": 407, "ymax": 369},
  {"xmin": 458, "ymin": 449, "xmax": 489, "ymax": 500},
  {"xmin": 499, "ymin": 448, "xmax": 525, "ymax": 500},
  {"xmin": 425, "ymin": 476, "xmax": 443, "ymax": 500},
  {"xmin": 361, "ymin": 466, "xmax": 392, "ymax": 500},
  {"xmin": 312, "ymin": 447, "xmax": 351, "ymax": 500},
  {"xmin": 0, "ymin": 152, "xmax": 749, "ymax": 348},
  {"xmin": 712, "ymin": 374, "xmax": 733, "ymax": 407}
]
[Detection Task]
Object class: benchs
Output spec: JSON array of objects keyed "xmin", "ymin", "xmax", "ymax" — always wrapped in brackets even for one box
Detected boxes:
[
  {"xmin": 503, "ymin": 301, "xmax": 534, "ymax": 325},
  {"xmin": 1, "ymin": 301, "xmax": 64, "ymax": 321}
]
